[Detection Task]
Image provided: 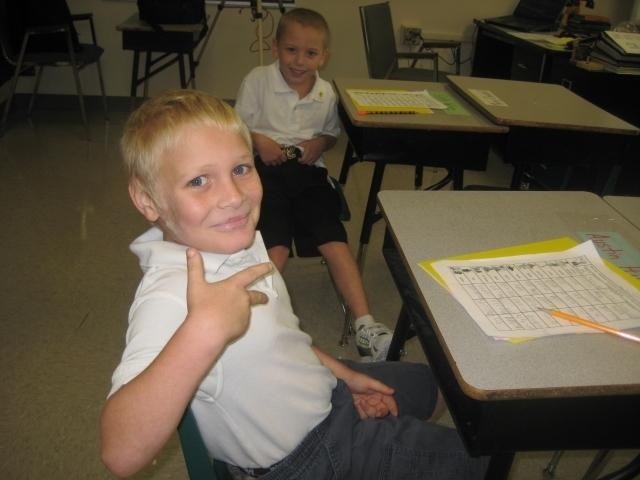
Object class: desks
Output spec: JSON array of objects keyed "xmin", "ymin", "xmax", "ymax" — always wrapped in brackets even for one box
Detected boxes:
[
  {"xmin": 111, "ymin": 9, "xmax": 209, "ymax": 126},
  {"xmin": 319, "ymin": 13, "xmax": 640, "ymax": 480}
]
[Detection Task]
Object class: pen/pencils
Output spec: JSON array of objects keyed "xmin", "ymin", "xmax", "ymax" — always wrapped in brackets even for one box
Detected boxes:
[
  {"xmin": 358, "ymin": 111, "xmax": 416, "ymax": 115},
  {"xmin": 538, "ymin": 308, "xmax": 640, "ymax": 342}
]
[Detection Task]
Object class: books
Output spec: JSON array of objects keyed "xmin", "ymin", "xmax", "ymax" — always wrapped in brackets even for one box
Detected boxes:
[{"xmin": 586, "ymin": 31, "xmax": 640, "ymax": 76}]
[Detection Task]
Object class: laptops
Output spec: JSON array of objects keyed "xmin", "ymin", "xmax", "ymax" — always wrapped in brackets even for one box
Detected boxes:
[{"xmin": 485, "ymin": 0, "xmax": 569, "ymax": 32}]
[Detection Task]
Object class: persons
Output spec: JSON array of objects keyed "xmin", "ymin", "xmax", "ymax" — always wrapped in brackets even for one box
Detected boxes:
[
  {"xmin": 233, "ymin": 7, "xmax": 407, "ymax": 363},
  {"xmin": 98, "ymin": 87, "xmax": 493, "ymax": 479}
]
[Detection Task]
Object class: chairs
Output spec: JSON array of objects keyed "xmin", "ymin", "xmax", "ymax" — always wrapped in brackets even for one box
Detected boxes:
[
  {"xmin": 1, "ymin": 0, "xmax": 112, "ymax": 144},
  {"xmin": 358, "ymin": 2, "xmax": 464, "ymax": 88},
  {"xmin": 172, "ymin": 398, "xmax": 239, "ymax": 480}
]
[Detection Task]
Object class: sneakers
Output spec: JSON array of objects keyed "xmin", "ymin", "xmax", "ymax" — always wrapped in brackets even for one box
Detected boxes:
[{"xmin": 353, "ymin": 318, "xmax": 406, "ymax": 365}]
